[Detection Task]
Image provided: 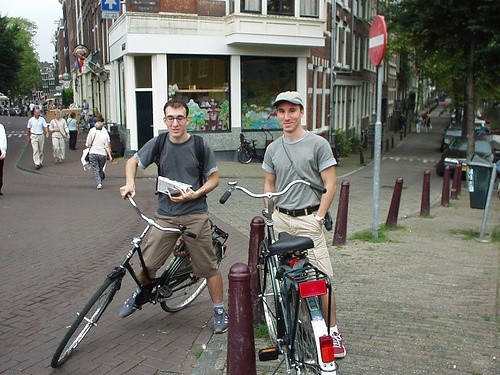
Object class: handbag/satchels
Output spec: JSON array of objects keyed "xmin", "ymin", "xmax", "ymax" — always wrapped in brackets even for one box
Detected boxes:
[
  {"xmin": 85, "ymin": 152, "xmax": 89, "ymax": 161},
  {"xmin": 324, "ymin": 212, "xmax": 332, "ymax": 231}
]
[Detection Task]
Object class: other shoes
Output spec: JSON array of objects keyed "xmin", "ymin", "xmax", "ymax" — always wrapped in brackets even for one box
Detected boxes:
[
  {"xmin": 96, "ymin": 184, "xmax": 102, "ymax": 189},
  {"xmin": 36, "ymin": 165, "xmax": 39, "ymax": 170},
  {"xmin": 59, "ymin": 159, "xmax": 62, "ymax": 163},
  {"xmin": 40, "ymin": 164, "xmax": 43, "ymax": 167},
  {"xmin": 54, "ymin": 158, "xmax": 58, "ymax": 163}
]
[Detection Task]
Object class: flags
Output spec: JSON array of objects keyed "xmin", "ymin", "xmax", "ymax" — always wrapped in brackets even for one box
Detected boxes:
[{"xmin": 74, "ymin": 53, "xmax": 85, "ymax": 73}]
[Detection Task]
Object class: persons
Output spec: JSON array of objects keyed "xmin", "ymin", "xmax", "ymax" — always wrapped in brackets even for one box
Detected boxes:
[
  {"xmin": 81, "ymin": 99, "xmax": 101, "ymax": 131},
  {"xmin": 120, "ymin": 99, "xmax": 228, "ymax": 333},
  {"xmin": 416, "ymin": 112, "xmax": 432, "ymax": 133},
  {"xmin": 26, "ymin": 108, "xmax": 50, "ymax": 171},
  {"xmin": 47, "ymin": 111, "xmax": 71, "ymax": 164},
  {"xmin": 84, "ymin": 115, "xmax": 114, "ymax": 189},
  {"xmin": 0, "ymin": 95, "xmax": 54, "ymax": 118},
  {"xmin": 66, "ymin": 112, "xmax": 79, "ymax": 150},
  {"xmin": 259, "ymin": 92, "xmax": 346, "ymax": 360},
  {"xmin": 0, "ymin": 123, "xmax": 8, "ymax": 196}
]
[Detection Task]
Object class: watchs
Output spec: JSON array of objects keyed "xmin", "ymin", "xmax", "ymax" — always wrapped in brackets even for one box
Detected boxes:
[{"xmin": 315, "ymin": 214, "xmax": 326, "ymax": 225}]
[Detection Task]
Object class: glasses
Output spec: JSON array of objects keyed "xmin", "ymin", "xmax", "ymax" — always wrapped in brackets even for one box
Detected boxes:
[{"xmin": 165, "ymin": 115, "xmax": 187, "ymax": 123}]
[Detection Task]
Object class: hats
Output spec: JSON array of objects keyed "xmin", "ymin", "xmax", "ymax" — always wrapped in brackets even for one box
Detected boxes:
[{"xmin": 273, "ymin": 91, "xmax": 304, "ymax": 109}]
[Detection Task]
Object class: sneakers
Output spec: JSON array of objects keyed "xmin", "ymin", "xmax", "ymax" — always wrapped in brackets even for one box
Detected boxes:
[
  {"xmin": 213, "ymin": 307, "xmax": 228, "ymax": 334},
  {"xmin": 119, "ymin": 289, "xmax": 140, "ymax": 317},
  {"xmin": 329, "ymin": 332, "xmax": 346, "ymax": 358}
]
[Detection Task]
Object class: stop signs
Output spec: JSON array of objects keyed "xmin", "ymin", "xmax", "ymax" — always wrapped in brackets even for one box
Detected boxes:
[{"xmin": 369, "ymin": 15, "xmax": 387, "ymax": 68}]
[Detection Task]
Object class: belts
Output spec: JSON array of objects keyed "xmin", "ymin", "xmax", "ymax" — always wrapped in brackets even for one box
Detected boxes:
[
  {"xmin": 32, "ymin": 133, "xmax": 41, "ymax": 135},
  {"xmin": 276, "ymin": 204, "xmax": 320, "ymax": 217}
]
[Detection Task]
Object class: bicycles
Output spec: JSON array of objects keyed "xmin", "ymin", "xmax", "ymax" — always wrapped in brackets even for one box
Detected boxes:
[
  {"xmin": 237, "ymin": 127, "xmax": 276, "ymax": 164},
  {"xmin": 50, "ymin": 191, "xmax": 229, "ymax": 369},
  {"xmin": 218, "ymin": 179, "xmax": 340, "ymax": 375}
]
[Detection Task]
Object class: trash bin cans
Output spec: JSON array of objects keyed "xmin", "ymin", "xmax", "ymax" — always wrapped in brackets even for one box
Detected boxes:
[{"xmin": 467, "ymin": 155, "xmax": 493, "ymax": 208}]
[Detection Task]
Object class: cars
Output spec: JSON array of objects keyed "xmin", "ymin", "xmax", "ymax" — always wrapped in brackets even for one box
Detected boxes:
[
  {"xmin": 439, "ymin": 107, "xmax": 490, "ymax": 152},
  {"xmin": 435, "ymin": 137, "xmax": 494, "ymax": 180},
  {"xmin": 12, "ymin": 105, "xmax": 27, "ymax": 116}
]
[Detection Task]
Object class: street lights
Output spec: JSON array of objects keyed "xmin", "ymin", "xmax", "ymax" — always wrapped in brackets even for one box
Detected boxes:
[{"xmin": 8, "ymin": 90, "xmax": 12, "ymax": 116}]
